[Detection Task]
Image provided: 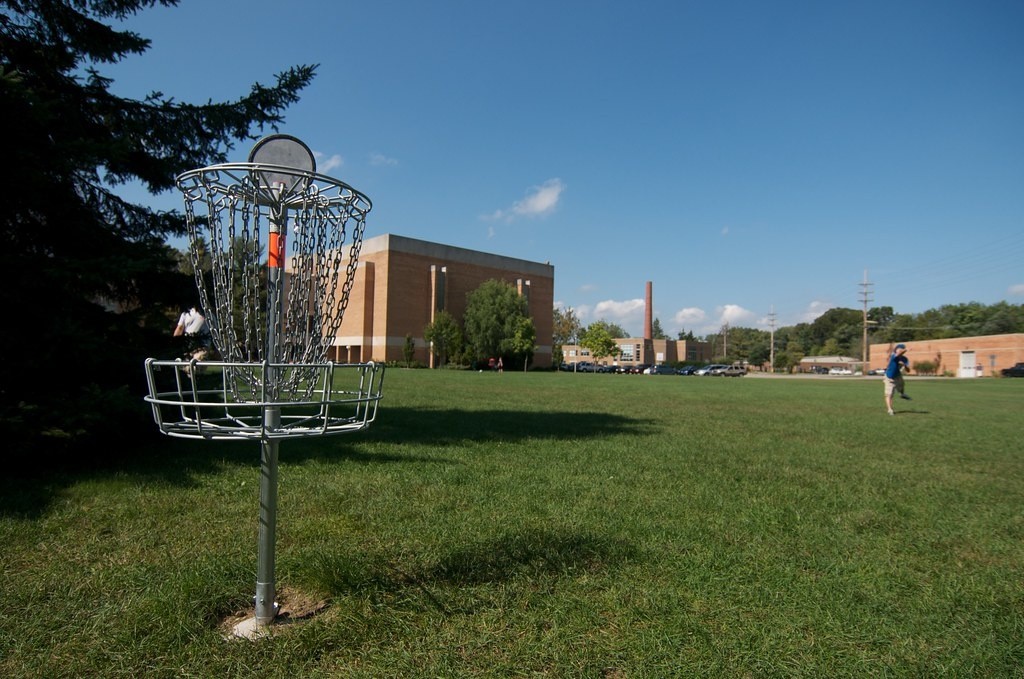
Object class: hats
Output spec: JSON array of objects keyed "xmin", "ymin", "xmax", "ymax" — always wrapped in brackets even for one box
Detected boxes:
[{"xmin": 894, "ymin": 343, "xmax": 905, "ymax": 353}]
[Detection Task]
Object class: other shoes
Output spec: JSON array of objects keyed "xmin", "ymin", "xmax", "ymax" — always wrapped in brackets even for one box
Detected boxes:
[
  {"xmin": 888, "ymin": 409, "xmax": 894, "ymax": 415},
  {"xmin": 900, "ymin": 393, "xmax": 911, "ymax": 400}
]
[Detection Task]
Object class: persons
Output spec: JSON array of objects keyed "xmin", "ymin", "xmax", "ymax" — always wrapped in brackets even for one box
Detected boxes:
[
  {"xmin": 883, "ymin": 345, "xmax": 912, "ymax": 416},
  {"xmin": 174, "ymin": 307, "xmax": 214, "ymax": 376}
]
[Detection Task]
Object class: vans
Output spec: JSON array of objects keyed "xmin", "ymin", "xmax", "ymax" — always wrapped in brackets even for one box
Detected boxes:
[
  {"xmin": 697, "ymin": 365, "xmax": 724, "ymax": 376},
  {"xmin": 713, "ymin": 366, "xmax": 747, "ymax": 377}
]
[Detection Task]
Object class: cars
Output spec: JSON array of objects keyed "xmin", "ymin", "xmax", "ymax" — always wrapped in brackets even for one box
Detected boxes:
[
  {"xmin": 630, "ymin": 364, "xmax": 650, "ymax": 374},
  {"xmin": 678, "ymin": 366, "xmax": 696, "ymax": 376},
  {"xmin": 561, "ymin": 360, "xmax": 629, "ymax": 373},
  {"xmin": 650, "ymin": 365, "xmax": 676, "ymax": 376}
]
